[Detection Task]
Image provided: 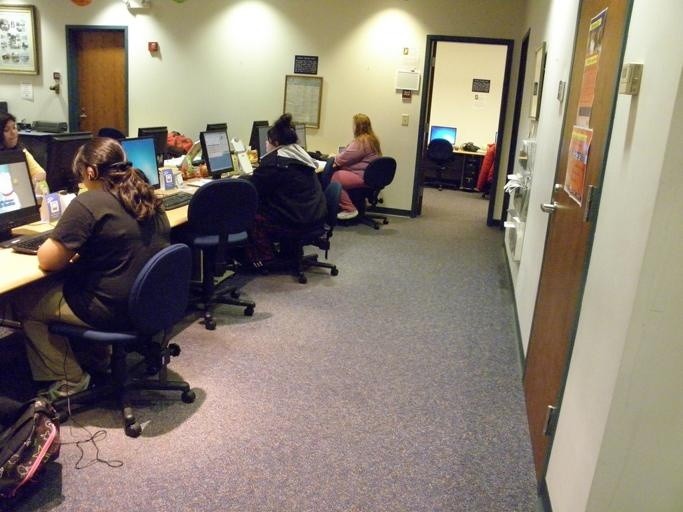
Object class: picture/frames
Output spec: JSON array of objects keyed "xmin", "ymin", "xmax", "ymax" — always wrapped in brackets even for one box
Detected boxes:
[
  {"xmin": 528, "ymin": 40, "xmax": 546, "ymax": 120},
  {"xmin": 282, "ymin": 74, "xmax": 322, "ymax": 130},
  {"xmin": 0, "ymin": 4, "xmax": 41, "ymax": 76}
]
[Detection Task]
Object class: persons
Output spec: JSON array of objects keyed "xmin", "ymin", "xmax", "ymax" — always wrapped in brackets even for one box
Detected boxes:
[
  {"xmin": 329, "ymin": 114, "xmax": 383, "ymax": 219},
  {"xmin": 12, "ymin": 136, "xmax": 172, "ymax": 408},
  {"xmin": 1, "ymin": 112, "xmax": 47, "ymax": 191},
  {"xmin": 233, "ymin": 114, "xmax": 327, "ymax": 271}
]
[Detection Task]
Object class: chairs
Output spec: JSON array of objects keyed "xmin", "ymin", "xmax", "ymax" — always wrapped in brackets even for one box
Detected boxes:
[
  {"xmin": 332, "ymin": 157, "xmax": 397, "ymax": 230},
  {"xmin": 318, "ymin": 155, "xmax": 334, "ymax": 191},
  {"xmin": 174, "ymin": 177, "xmax": 258, "ymax": 330},
  {"xmin": 424, "ymin": 137, "xmax": 457, "ymax": 191},
  {"xmin": 255, "ymin": 180, "xmax": 342, "ymax": 283},
  {"xmin": 47, "ymin": 241, "xmax": 195, "ymax": 437}
]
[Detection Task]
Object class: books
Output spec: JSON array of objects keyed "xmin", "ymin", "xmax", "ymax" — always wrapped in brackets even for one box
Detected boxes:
[{"xmin": 187, "ymin": 177, "xmax": 214, "ymax": 187}]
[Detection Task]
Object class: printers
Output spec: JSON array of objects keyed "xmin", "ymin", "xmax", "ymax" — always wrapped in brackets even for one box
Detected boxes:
[{"xmin": 32, "ymin": 120, "xmax": 68, "ymax": 133}]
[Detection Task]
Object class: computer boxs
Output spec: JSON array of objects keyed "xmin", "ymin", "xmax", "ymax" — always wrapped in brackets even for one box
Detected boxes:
[
  {"xmin": 463, "ymin": 157, "xmax": 481, "ymax": 188},
  {"xmin": 0, "ymin": 325, "xmax": 35, "ymax": 425}
]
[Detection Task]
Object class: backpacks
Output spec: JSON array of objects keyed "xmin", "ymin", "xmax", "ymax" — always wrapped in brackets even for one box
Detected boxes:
[{"xmin": 0, "ymin": 397, "xmax": 60, "ymax": 510}]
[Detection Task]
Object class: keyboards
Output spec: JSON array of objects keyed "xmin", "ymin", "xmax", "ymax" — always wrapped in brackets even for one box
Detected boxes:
[
  {"xmin": 320, "ymin": 154, "xmax": 334, "ymax": 161},
  {"xmin": 12, "ymin": 229, "xmax": 56, "ymax": 254},
  {"xmin": 160, "ymin": 191, "xmax": 193, "ymax": 210},
  {"xmin": 176, "ymin": 160, "xmax": 203, "ymax": 168}
]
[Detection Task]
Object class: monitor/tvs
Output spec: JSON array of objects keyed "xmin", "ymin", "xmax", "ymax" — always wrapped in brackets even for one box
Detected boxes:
[
  {"xmin": 249, "ymin": 121, "xmax": 269, "ymax": 150},
  {"xmin": 431, "ymin": 126, "xmax": 457, "ymax": 145},
  {"xmin": 0, "ymin": 151, "xmax": 41, "ymax": 248},
  {"xmin": 46, "ymin": 131, "xmax": 93, "ymax": 195},
  {"xmin": 294, "ymin": 122, "xmax": 306, "ymax": 152},
  {"xmin": 255, "ymin": 126, "xmax": 273, "ymax": 164},
  {"xmin": 202, "ymin": 123, "xmax": 227, "ymax": 160},
  {"xmin": 118, "ymin": 136, "xmax": 160, "ymax": 189},
  {"xmin": 200, "ymin": 129, "xmax": 234, "ymax": 179},
  {"xmin": 138, "ymin": 126, "xmax": 168, "ymax": 167}
]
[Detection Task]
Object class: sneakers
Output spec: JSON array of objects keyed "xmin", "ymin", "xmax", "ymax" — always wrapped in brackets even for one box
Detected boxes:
[
  {"xmin": 238, "ymin": 260, "xmax": 265, "ymax": 273},
  {"xmin": 38, "ymin": 371, "xmax": 92, "ymax": 402},
  {"xmin": 337, "ymin": 209, "xmax": 358, "ymax": 220}
]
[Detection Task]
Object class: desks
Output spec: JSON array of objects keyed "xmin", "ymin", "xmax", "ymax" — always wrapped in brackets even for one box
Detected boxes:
[
  {"xmin": 0, "ymin": 129, "xmax": 77, "ymax": 191},
  {"xmin": 0, "ymin": 155, "xmax": 329, "ymax": 322},
  {"xmin": 425, "ymin": 148, "xmax": 487, "ymax": 192}
]
